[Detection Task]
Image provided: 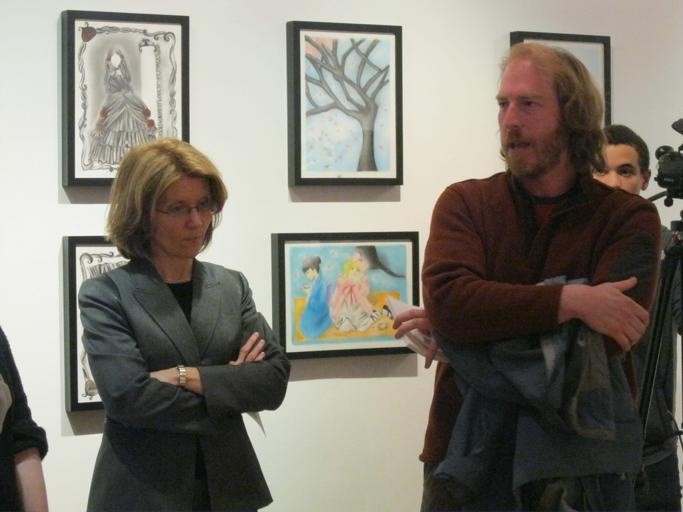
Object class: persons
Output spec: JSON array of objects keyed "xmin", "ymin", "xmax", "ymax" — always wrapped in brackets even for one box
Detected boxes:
[
  {"xmin": 392, "ymin": 41, "xmax": 662, "ymax": 511},
  {"xmin": 0, "ymin": 330, "xmax": 48, "ymax": 512},
  {"xmin": 78, "ymin": 137, "xmax": 291, "ymax": 510},
  {"xmin": 586, "ymin": 124, "xmax": 683, "ymax": 512}
]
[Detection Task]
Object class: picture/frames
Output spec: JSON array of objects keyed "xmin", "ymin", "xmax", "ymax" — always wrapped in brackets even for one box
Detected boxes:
[
  {"xmin": 510, "ymin": 31, "xmax": 611, "ymax": 134},
  {"xmin": 62, "ymin": 235, "xmax": 132, "ymax": 413},
  {"xmin": 286, "ymin": 20, "xmax": 404, "ymax": 186},
  {"xmin": 271, "ymin": 231, "xmax": 420, "ymax": 360},
  {"xmin": 61, "ymin": 10, "xmax": 190, "ymax": 186}
]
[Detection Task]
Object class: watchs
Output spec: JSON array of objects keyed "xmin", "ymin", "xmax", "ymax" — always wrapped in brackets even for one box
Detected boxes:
[{"xmin": 178, "ymin": 364, "xmax": 186, "ymax": 388}]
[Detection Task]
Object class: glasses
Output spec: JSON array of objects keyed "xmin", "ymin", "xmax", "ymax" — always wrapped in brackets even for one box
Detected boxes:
[{"xmin": 155, "ymin": 200, "xmax": 218, "ymax": 218}]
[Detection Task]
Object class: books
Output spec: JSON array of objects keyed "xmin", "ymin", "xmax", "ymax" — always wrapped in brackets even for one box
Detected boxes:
[{"xmin": 383, "ymin": 297, "xmax": 453, "ymax": 363}]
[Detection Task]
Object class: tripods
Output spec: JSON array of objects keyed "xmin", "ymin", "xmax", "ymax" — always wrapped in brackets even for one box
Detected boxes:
[{"xmin": 640, "ymin": 239, "xmax": 683, "ymax": 432}]
